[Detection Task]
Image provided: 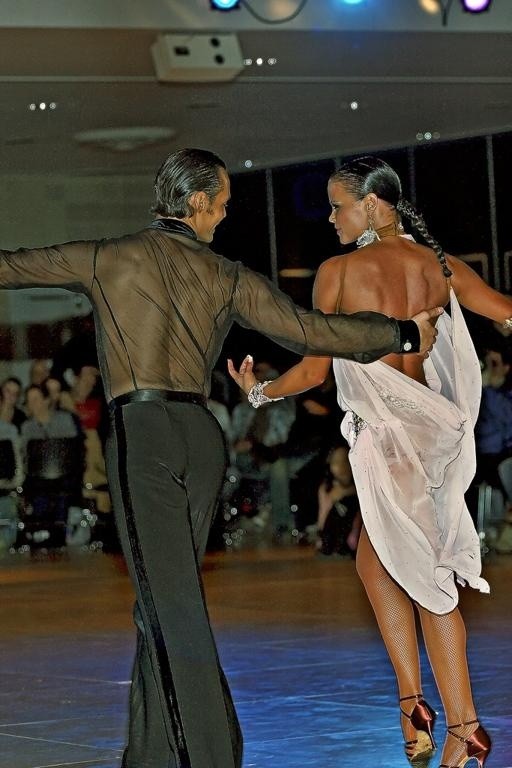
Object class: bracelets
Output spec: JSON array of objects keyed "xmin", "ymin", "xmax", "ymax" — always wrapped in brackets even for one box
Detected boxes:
[
  {"xmin": 246, "ymin": 381, "xmax": 277, "ymax": 409},
  {"xmin": 502, "ymin": 315, "xmax": 512, "ymax": 329}
]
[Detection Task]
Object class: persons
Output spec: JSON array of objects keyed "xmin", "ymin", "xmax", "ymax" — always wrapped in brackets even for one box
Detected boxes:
[
  {"xmin": 475, "ymin": 348, "xmax": 512, "ymax": 523},
  {"xmin": 1, "ymin": 147, "xmax": 446, "ymax": 767},
  {"xmin": 207, "ymin": 362, "xmax": 362, "ymax": 557},
  {"xmin": 3, "ymin": 343, "xmax": 123, "ymax": 558},
  {"xmin": 221, "ymin": 153, "xmax": 512, "ymax": 767}
]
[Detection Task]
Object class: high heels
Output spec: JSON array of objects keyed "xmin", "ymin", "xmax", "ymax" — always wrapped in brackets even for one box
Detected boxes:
[
  {"xmin": 439, "ymin": 718, "xmax": 493, "ymax": 768},
  {"xmin": 397, "ymin": 694, "xmax": 438, "ymax": 768}
]
